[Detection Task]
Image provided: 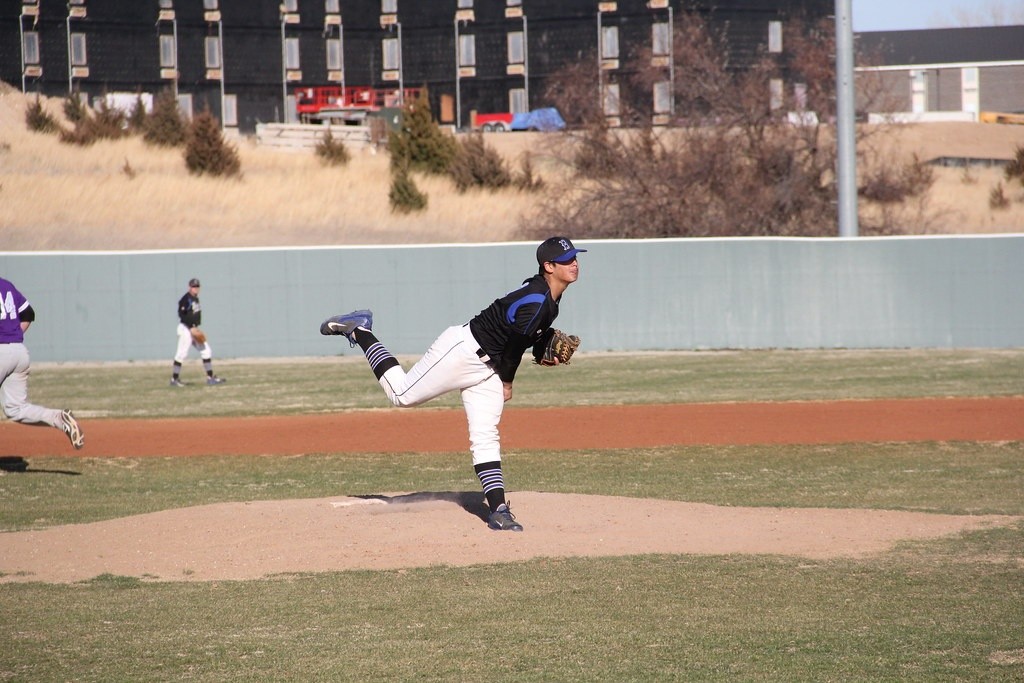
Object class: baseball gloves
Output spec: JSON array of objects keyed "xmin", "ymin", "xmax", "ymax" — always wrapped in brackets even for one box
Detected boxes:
[
  {"xmin": 540, "ymin": 329, "xmax": 581, "ymax": 366},
  {"xmin": 194, "ymin": 329, "xmax": 206, "ymax": 343}
]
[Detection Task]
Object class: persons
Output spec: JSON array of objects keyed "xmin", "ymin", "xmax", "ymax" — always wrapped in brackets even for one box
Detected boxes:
[
  {"xmin": 171, "ymin": 279, "xmax": 226, "ymax": 387},
  {"xmin": 0, "ymin": 278, "xmax": 85, "ymax": 450},
  {"xmin": 320, "ymin": 237, "xmax": 588, "ymax": 531}
]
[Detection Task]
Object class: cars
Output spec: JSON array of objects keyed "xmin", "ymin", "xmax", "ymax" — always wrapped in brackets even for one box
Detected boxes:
[{"xmin": 806, "ymin": 91, "xmax": 864, "ymax": 125}]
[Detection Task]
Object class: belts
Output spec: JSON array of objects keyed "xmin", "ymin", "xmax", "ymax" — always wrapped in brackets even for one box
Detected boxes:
[{"xmin": 462, "ymin": 323, "xmax": 492, "ymax": 365}]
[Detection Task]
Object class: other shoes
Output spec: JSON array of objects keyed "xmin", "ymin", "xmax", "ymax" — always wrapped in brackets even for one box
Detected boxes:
[
  {"xmin": 206, "ymin": 375, "xmax": 225, "ymax": 385},
  {"xmin": 169, "ymin": 377, "xmax": 184, "ymax": 388}
]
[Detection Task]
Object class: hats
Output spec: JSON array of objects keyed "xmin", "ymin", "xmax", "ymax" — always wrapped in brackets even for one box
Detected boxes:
[
  {"xmin": 536, "ymin": 237, "xmax": 586, "ymax": 264},
  {"xmin": 189, "ymin": 279, "xmax": 200, "ymax": 288}
]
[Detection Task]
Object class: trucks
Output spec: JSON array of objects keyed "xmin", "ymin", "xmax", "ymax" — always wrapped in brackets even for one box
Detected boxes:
[{"xmin": 294, "ymin": 86, "xmax": 429, "ymax": 126}]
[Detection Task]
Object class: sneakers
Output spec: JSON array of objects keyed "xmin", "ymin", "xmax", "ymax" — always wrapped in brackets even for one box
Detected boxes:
[
  {"xmin": 320, "ymin": 309, "xmax": 373, "ymax": 348},
  {"xmin": 488, "ymin": 501, "xmax": 522, "ymax": 531},
  {"xmin": 60, "ymin": 410, "xmax": 84, "ymax": 449}
]
